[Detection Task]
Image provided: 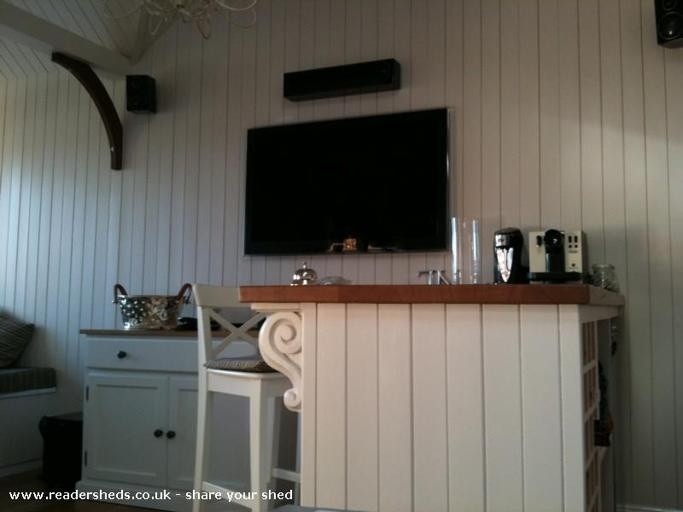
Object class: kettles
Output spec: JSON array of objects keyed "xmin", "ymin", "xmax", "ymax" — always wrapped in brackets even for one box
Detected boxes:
[{"xmin": 493, "ymin": 227, "xmax": 530, "ymax": 284}]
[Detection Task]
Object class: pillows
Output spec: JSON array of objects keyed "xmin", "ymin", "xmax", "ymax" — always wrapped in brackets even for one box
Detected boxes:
[{"xmin": 1, "ymin": 314, "xmax": 34, "ymax": 369}]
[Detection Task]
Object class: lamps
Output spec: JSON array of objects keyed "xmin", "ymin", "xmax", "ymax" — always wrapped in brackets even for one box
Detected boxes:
[{"xmin": 102, "ymin": 0, "xmax": 259, "ymax": 41}]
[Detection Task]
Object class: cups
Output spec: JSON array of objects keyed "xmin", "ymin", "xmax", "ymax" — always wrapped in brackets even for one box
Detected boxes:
[{"xmin": 451, "ymin": 217, "xmax": 482, "ymax": 285}]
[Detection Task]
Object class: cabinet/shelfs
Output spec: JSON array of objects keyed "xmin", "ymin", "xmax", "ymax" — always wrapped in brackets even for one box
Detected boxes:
[
  {"xmin": 76, "ymin": 326, "xmax": 274, "ymax": 512},
  {"xmin": 238, "ymin": 284, "xmax": 625, "ymax": 512}
]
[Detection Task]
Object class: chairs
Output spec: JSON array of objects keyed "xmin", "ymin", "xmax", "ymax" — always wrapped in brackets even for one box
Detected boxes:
[{"xmin": 187, "ymin": 281, "xmax": 294, "ymax": 511}]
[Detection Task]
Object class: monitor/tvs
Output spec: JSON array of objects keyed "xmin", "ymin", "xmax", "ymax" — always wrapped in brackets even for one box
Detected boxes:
[{"xmin": 246, "ymin": 107, "xmax": 449, "ymax": 256}]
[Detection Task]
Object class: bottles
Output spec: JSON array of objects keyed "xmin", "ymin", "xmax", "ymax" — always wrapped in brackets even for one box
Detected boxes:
[{"xmin": 591, "ymin": 264, "xmax": 617, "ymax": 292}]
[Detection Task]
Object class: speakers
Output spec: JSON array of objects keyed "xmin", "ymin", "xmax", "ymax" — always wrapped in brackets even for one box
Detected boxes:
[
  {"xmin": 128, "ymin": 75, "xmax": 157, "ymax": 114},
  {"xmin": 283, "ymin": 58, "xmax": 401, "ymax": 103},
  {"xmin": 654, "ymin": 1, "xmax": 683, "ymax": 48}
]
